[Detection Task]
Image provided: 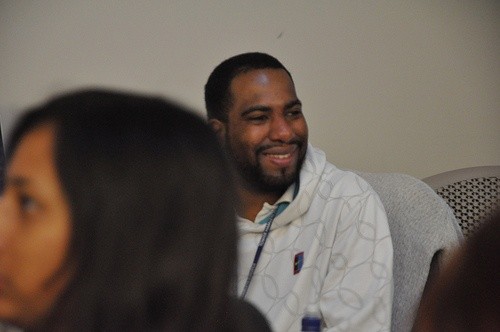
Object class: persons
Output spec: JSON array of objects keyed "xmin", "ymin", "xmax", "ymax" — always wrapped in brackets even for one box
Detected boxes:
[
  {"xmin": 204, "ymin": 52, "xmax": 393, "ymax": 332},
  {"xmin": 0, "ymin": 88, "xmax": 273, "ymax": 332},
  {"xmin": 413, "ymin": 200, "xmax": 500, "ymax": 332}
]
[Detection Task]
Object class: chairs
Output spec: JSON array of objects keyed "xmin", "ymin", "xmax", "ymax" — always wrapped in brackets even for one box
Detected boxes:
[
  {"xmin": 422, "ymin": 166, "xmax": 500, "ymax": 239},
  {"xmin": 351, "ymin": 171, "xmax": 466, "ymax": 332}
]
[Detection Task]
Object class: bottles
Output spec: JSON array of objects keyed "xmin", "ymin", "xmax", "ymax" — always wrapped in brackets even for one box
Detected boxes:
[{"xmin": 302, "ymin": 302, "xmax": 321, "ymax": 332}]
